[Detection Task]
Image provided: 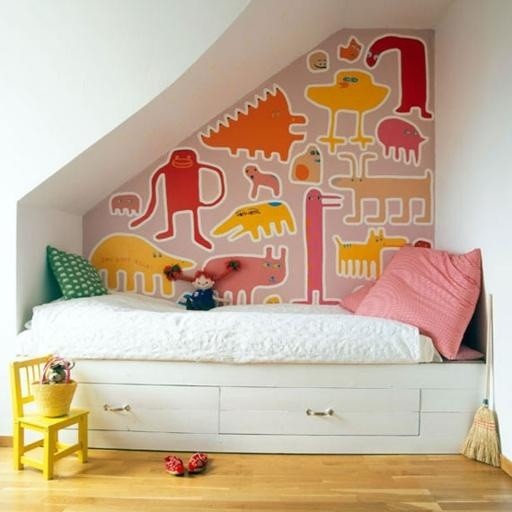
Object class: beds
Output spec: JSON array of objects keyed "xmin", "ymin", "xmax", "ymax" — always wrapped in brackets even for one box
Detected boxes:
[{"xmin": 16, "ymin": 292, "xmax": 490, "ymax": 455}]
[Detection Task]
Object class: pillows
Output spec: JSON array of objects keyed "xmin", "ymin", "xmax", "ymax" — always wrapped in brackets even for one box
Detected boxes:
[
  {"xmin": 46, "ymin": 245, "xmax": 110, "ymax": 300},
  {"xmin": 340, "ymin": 280, "xmax": 375, "ymax": 312},
  {"xmin": 355, "ymin": 244, "xmax": 482, "ymax": 359}
]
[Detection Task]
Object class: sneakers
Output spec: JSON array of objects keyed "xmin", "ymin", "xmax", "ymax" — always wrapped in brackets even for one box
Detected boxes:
[
  {"xmin": 186, "ymin": 452, "xmax": 207, "ymax": 472},
  {"xmin": 164, "ymin": 456, "xmax": 184, "ymax": 475}
]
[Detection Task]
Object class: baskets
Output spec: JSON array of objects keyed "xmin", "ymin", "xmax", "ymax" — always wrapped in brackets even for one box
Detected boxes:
[{"xmin": 29, "ymin": 355, "xmax": 77, "ymax": 418}]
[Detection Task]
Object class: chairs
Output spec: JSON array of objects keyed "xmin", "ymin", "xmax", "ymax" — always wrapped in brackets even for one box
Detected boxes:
[{"xmin": 12, "ymin": 354, "xmax": 90, "ymax": 479}]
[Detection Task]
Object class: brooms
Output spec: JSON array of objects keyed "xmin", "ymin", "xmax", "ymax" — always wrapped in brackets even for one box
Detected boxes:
[{"xmin": 460, "ymin": 295, "xmax": 501, "ymax": 467}]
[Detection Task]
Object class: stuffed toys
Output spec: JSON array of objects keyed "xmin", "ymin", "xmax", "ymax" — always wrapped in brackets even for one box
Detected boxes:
[{"xmin": 164, "ymin": 260, "xmax": 241, "ymax": 310}]
